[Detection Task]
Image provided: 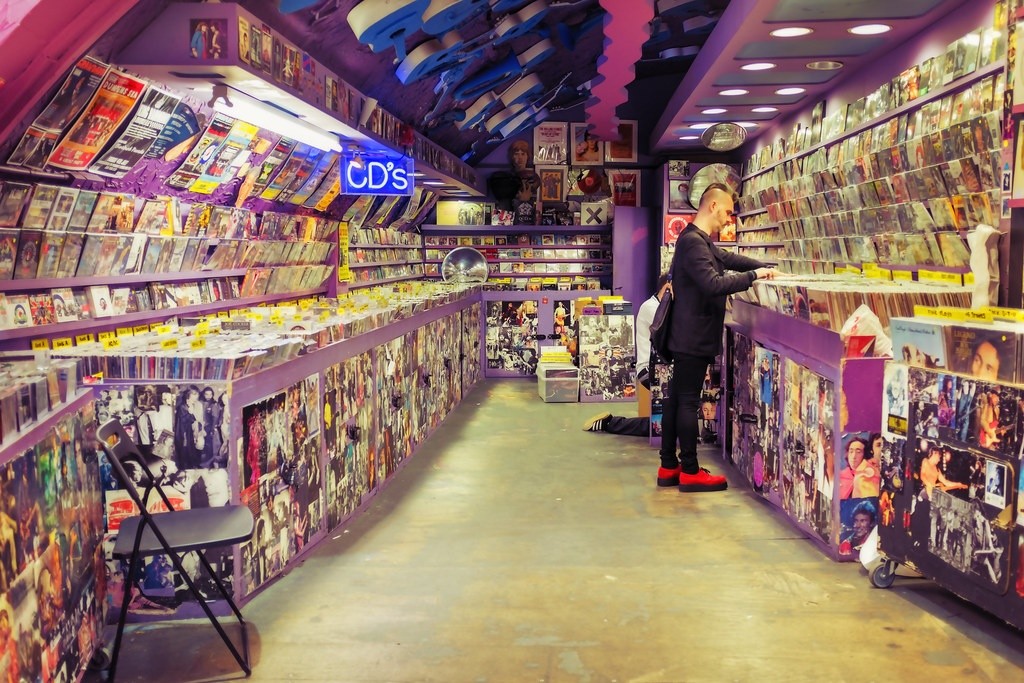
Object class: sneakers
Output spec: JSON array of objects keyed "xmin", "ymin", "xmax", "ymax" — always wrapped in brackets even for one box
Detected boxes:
[
  {"xmin": 582, "ymin": 410, "xmax": 613, "ymax": 432},
  {"xmin": 656, "ymin": 462, "xmax": 682, "ymax": 487},
  {"xmin": 678, "ymin": 465, "xmax": 728, "ymax": 492}
]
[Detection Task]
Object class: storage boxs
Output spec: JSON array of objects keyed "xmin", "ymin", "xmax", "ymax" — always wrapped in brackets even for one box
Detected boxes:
[
  {"xmin": 582, "ymin": 306, "xmax": 602, "ymax": 315},
  {"xmin": 574, "ymin": 299, "xmax": 604, "ymax": 322},
  {"xmin": 603, "ymin": 300, "xmax": 633, "ymax": 315},
  {"xmin": 536, "ymin": 369, "xmax": 581, "ymax": 403}
]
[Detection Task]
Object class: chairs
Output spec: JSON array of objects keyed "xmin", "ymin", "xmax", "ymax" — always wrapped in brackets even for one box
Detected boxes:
[{"xmin": 95, "ymin": 416, "xmax": 257, "ymax": 683}]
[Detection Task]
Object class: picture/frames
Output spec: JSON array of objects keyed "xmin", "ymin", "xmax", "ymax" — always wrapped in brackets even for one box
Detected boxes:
[
  {"xmin": 425, "ymin": 234, "xmax": 605, "ymax": 290},
  {"xmin": 533, "ymin": 122, "xmax": 568, "ymax": 165},
  {"xmin": 535, "ymin": 165, "xmax": 568, "ymax": 202},
  {"xmin": 605, "ymin": 120, "xmax": 637, "ymax": 163},
  {"xmin": 570, "ymin": 123, "xmax": 604, "ymax": 165},
  {"xmin": 608, "ymin": 170, "xmax": 641, "ymax": 207},
  {"xmin": 1000, "ymin": 196, "xmax": 1012, "ymax": 219},
  {"xmin": 1001, "ymin": 170, "xmax": 1012, "ymax": 194}
]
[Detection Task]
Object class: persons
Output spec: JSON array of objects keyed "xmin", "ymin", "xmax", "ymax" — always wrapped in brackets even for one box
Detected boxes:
[
  {"xmin": 582, "ymin": 273, "xmax": 677, "ymax": 436},
  {"xmin": 733, "ymin": 37, "xmax": 1024, "ymax": 609},
  {"xmin": 657, "ymin": 184, "xmax": 776, "ymax": 492}
]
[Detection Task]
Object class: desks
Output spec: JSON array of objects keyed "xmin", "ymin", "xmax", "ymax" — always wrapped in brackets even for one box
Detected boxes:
[
  {"xmin": 872, "ymin": 360, "xmax": 1024, "ymax": 632},
  {"xmin": 482, "ymin": 290, "xmax": 611, "ymax": 378},
  {"xmin": 714, "ymin": 299, "xmax": 893, "ymax": 564},
  {"xmin": 100, "ymin": 292, "xmax": 484, "ymax": 625},
  {"xmin": 0, "ymin": 386, "xmax": 114, "ymax": 683}
]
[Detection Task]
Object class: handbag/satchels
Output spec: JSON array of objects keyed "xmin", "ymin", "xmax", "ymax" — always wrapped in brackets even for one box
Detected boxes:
[{"xmin": 648, "ymin": 274, "xmax": 673, "ymax": 366}]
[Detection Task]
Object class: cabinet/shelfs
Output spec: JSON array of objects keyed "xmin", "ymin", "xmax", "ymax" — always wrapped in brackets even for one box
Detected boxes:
[
  {"xmin": 424, "ymin": 245, "xmax": 613, "ymax": 277},
  {"xmin": 349, "ymin": 244, "xmax": 425, "ymax": 289}
]
